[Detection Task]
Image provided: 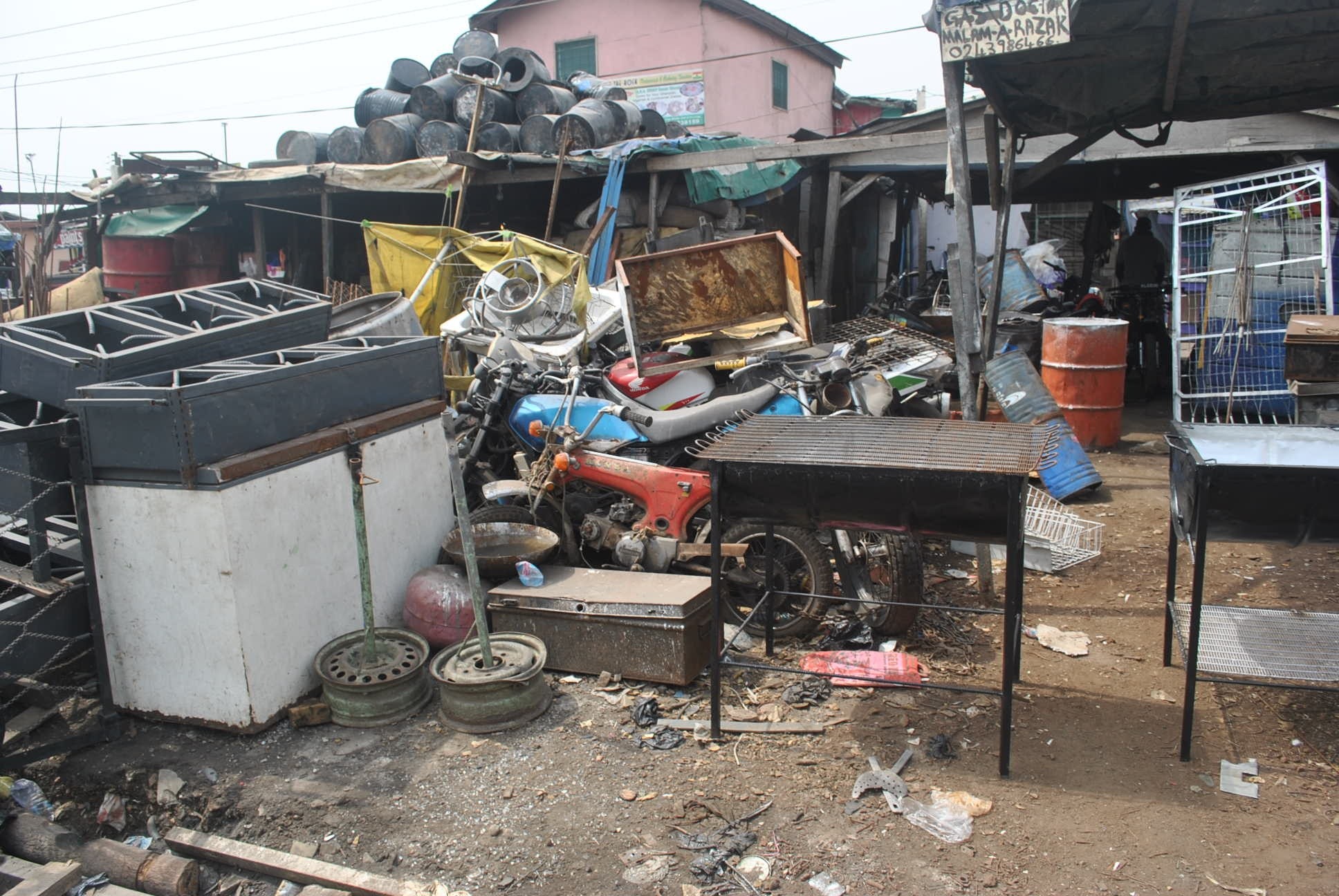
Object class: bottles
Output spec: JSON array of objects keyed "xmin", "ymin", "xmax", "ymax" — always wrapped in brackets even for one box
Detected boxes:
[{"xmin": 10, "ymin": 778, "xmax": 54, "ymax": 821}]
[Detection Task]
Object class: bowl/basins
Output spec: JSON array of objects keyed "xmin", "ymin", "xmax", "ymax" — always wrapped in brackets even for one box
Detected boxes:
[{"xmin": 441, "ymin": 522, "xmax": 560, "ymax": 582}]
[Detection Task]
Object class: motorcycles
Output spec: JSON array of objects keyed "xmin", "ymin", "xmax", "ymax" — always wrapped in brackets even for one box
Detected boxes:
[
  {"xmin": 857, "ymin": 269, "xmax": 958, "ymax": 350},
  {"xmin": 446, "ymin": 320, "xmax": 923, "ymax": 640}
]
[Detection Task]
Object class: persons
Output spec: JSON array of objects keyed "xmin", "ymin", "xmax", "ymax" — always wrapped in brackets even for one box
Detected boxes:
[
  {"xmin": 0, "ymin": 251, "xmax": 19, "ymax": 289},
  {"xmin": 1081, "ymin": 201, "xmax": 1167, "ymax": 399}
]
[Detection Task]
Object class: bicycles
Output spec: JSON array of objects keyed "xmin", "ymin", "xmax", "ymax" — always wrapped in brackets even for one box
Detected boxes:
[{"xmin": 1103, "ymin": 277, "xmax": 1174, "ymax": 397}]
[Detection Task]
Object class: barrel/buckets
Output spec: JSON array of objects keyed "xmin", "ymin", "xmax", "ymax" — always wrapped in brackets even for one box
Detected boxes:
[
  {"xmin": 978, "ymin": 250, "xmax": 1051, "ymax": 313},
  {"xmin": 363, "ymin": 113, "xmax": 425, "ymax": 165},
  {"xmin": 173, "ymin": 226, "xmax": 242, "ymax": 291},
  {"xmin": 984, "ymin": 349, "xmax": 1102, "ymax": 501},
  {"xmin": 518, "ymin": 113, "xmax": 563, "ymax": 154},
  {"xmin": 993, "ymin": 310, "xmax": 1042, "ymax": 373},
  {"xmin": 451, "ymin": 83, "xmax": 515, "ymax": 130},
  {"xmin": 410, "ymin": 74, "xmax": 467, "ymax": 122},
  {"xmin": 275, "ymin": 130, "xmax": 297, "ymax": 158},
  {"xmin": 563, "ymin": 69, "xmax": 627, "ymax": 104},
  {"xmin": 640, "ymin": 110, "xmax": 666, "ymax": 138},
  {"xmin": 325, "ymin": 126, "xmax": 367, "ymax": 163},
  {"xmin": 1042, "ymin": 316, "xmax": 1129, "ymax": 452},
  {"xmin": 353, "ymin": 87, "xmax": 411, "ymax": 127},
  {"xmin": 415, "ymin": 120, "xmax": 469, "ymax": 159},
  {"xmin": 475, "ymin": 122, "xmax": 522, "ymax": 154},
  {"xmin": 429, "ymin": 54, "xmax": 457, "ymax": 79},
  {"xmin": 100, "ymin": 234, "xmax": 174, "ymax": 300},
  {"xmin": 514, "ymin": 83, "xmax": 577, "ymax": 122},
  {"xmin": 385, "ymin": 59, "xmax": 428, "ymax": 93},
  {"xmin": 285, "ymin": 130, "xmax": 330, "ymax": 164},
  {"xmin": 452, "ymin": 28, "xmax": 496, "ymax": 79},
  {"xmin": 602, "ymin": 99, "xmax": 643, "ymax": 142},
  {"xmin": 550, "ymin": 98, "xmax": 617, "ymax": 154}
]
